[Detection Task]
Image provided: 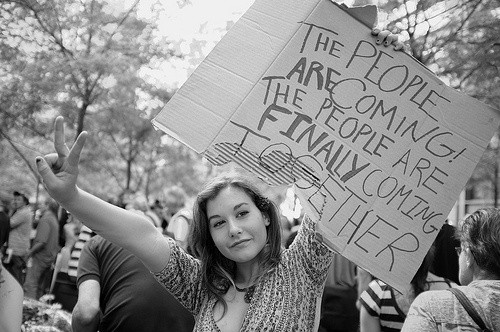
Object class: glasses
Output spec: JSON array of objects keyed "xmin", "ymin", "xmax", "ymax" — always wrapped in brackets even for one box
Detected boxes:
[{"xmin": 455, "ymin": 246, "xmax": 470, "ymax": 255}]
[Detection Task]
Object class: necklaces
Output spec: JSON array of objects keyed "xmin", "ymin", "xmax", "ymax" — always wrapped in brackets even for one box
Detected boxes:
[{"xmin": 232, "ymin": 280, "xmax": 255, "ymax": 304}]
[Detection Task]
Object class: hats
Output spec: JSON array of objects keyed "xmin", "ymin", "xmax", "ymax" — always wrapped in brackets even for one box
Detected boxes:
[{"xmin": 14, "ymin": 191, "xmax": 30, "ymax": 201}]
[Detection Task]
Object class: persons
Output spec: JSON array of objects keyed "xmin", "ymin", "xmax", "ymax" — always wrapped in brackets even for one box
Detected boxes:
[
  {"xmin": 71, "ymin": 232, "xmax": 195, "ymax": 332},
  {"xmin": 429, "ymin": 219, "xmax": 464, "ymax": 287},
  {"xmin": 0, "ymin": 181, "xmax": 203, "ymax": 314},
  {"xmin": 401, "ymin": 208, "xmax": 500, "ymax": 332},
  {"xmin": 317, "ymin": 251, "xmax": 374, "ymax": 332},
  {"xmin": 36, "ymin": 116, "xmax": 337, "ymax": 332},
  {"xmin": 356, "ymin": 243, "xmax": 462, "ymax": 332},
  {"xmin": 0, "ymin": 256, "xmax": 26, "ymax": 332}
]
